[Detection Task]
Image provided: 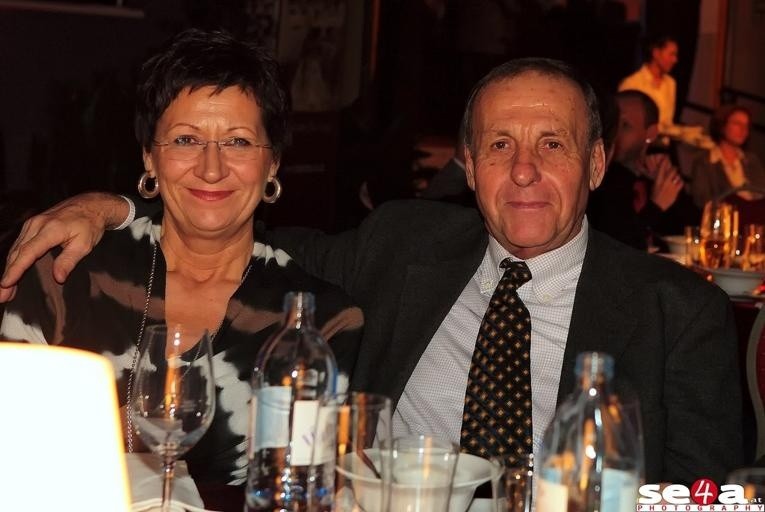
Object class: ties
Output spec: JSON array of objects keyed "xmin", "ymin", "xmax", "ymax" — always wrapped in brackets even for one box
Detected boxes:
[{"xmin": 460, "ymin": 260, "xmax": 533, "ymax": 499}]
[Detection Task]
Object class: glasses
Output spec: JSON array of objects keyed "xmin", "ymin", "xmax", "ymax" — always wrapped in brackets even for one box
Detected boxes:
[{"xmin": 153, "ymin": 136, "xmax": 272, "ymax": 160}]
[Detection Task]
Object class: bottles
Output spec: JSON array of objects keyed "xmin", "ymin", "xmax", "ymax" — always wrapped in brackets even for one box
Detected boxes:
[
  {"xmin": 244, "ymin": 292, "xmax": 338, "ymax": 512},
  {"xmin": 534, "ymin": 353, "xmax": 643, "ymax": 512}
]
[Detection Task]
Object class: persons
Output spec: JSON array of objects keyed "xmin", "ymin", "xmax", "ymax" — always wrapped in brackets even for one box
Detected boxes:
[
  {"xmin": 2, "ymin": 58, "xmax": 755, "ymax": 493},
  {"xmin": 579, "ymin": 88, "xmax": 702, "ymax": 256},
  {"xmin": 690, "ymin": 102, "xmax": 765, "ymax": 213},
  {"xmin": 616, "ymin": 29, "xmax": 703, "ymax": 148},
  {"xmin": 1, "ymin": 26, "xmax": 366, "ymax": 488},
  {"xmin": 423, "ymin": 141, "xmax": 478, "ymax": 213}
]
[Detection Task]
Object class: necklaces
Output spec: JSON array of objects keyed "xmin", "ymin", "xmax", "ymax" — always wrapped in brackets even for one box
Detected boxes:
[{"xmin": 121, "ymin": 239, "xmax": 265, "ymax": 456}]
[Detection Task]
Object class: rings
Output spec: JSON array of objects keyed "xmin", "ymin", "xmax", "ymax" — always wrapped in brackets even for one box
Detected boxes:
[{"xmin": 672, "ymin": 178, "xmax": 679, "ymax": 184}]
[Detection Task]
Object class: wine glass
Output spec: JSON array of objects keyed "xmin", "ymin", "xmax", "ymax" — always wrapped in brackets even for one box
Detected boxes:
[{"xmin": 130, "ymin": 325, "xmax": 216, "ymax": 512}]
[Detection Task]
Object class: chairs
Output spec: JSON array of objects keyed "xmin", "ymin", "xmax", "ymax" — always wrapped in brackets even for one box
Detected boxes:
[{"xmin": 730, "ymin": 304, "xmax": 765, "ymax": 467}]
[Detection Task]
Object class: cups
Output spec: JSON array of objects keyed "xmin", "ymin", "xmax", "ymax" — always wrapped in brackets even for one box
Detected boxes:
[
  {"xmin": 727, "ymin": 467, "xmax": 765, "ymax": 503},
  {"xmin": 684, "ymin": 201, "xmax": 765, "ymax": 273},
  {"xmin": 306, "ymin": 393, "xmax": 533, "ymax": 512}
]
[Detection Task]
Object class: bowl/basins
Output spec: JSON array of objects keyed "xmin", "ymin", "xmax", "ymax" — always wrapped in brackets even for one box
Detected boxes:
[
  {"xmin": 697, "ymin": 263, "xmax": 765, "ymax": 297},
  {"xmin": 659, "ymin": 233, "xmax": 692, "ymax": 254}
]
